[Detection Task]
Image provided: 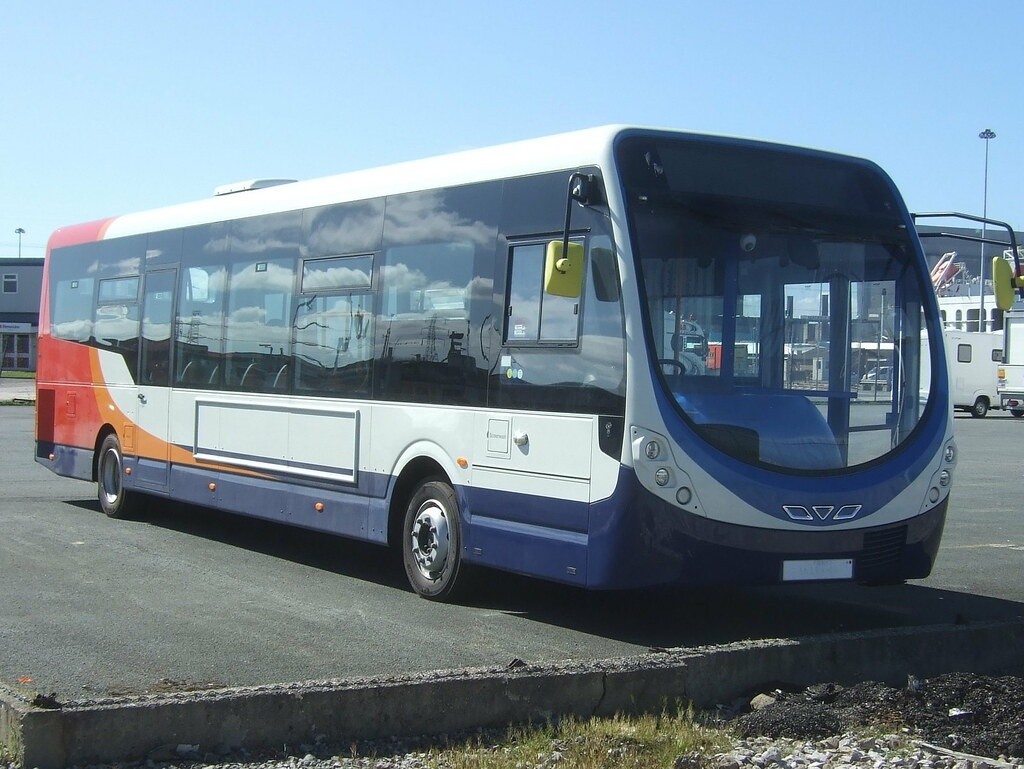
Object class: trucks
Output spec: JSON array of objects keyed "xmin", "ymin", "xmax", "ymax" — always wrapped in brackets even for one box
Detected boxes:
[
  {"xmin": 890, "ymin": 327, "xmax": 1001, "ymax": 418},
  {"xmin": 664, "ymin": 310, "xmax": 709, "ymax": 376},
  {"xmin": 996, "ymin": 300, "xmax": 1024, "ymax": 418}
]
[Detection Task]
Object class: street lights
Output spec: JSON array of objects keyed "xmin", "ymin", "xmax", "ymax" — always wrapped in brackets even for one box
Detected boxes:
[
  {"xmin": 15, "ymin": 228, "xmax": 25, "ymax": 259},
  {"xmin": 978, "ymin": 128, "xmax": 996, "ymax": 332}
]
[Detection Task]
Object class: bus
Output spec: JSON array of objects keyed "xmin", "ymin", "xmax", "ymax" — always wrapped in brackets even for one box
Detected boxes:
[{"xmin": 32, "ymin": 122, "xmax": 1024, "ymax": 601}]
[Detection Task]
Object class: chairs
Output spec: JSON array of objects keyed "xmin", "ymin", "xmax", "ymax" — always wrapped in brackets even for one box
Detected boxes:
[{"xmin": 148, "ymin": 360, "xmax": 292, "ymax": 390}]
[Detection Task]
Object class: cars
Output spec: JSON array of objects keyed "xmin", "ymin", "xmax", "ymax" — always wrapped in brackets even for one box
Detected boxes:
[{"xmin": 862, "ymin": 367, "xmax": 892, "ymax": 381}]
